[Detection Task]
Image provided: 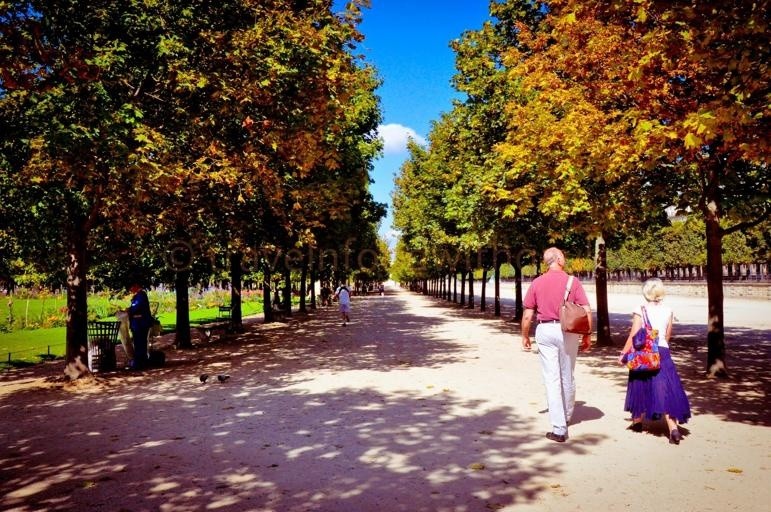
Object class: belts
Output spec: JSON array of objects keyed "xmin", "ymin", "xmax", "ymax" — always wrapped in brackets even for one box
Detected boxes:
[{"xmin": 537, "ymin": 317, "xmax": 562, "ymax": 325}]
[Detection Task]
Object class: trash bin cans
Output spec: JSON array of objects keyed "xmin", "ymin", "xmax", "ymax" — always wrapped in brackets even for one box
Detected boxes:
[{"xmin": 87, "ymin": 321, "xmax": 121, "ymax": 373}]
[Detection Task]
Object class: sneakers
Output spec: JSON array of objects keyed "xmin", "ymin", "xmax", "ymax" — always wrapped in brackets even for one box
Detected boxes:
[{"xmin": 546, "ymin": 433, "xmax": 565, "ymax": 443}]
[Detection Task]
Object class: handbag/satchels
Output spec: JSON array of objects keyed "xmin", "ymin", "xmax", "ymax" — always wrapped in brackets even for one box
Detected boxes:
[
  {"xmin": 559, "ymin": 275, "xmax": 590, "ymax": 334},
  {"xmin": 621, "ymin": 306, "xmax": 661, "ymax": 371}
]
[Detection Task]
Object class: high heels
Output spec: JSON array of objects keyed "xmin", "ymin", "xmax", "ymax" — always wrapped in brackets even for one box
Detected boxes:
[
  {"xmin": 629, "ymin": 422, "xmax": 643, "ymax": 431},
  {"xmin": 668, "ymin": 429, "xmax": 680, "ymax": 444}
]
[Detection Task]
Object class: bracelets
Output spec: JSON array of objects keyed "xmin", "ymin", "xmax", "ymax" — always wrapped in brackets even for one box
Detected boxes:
[
  {"xmin": 618, "ymin": 351, "xmax": 625, "ymax": 356},
  {"xmin": 587, "ymin": 332, "xmax": 592, "ymax": 336}
]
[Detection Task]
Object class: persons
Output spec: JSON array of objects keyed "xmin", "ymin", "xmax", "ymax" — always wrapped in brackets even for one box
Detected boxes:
[
  {"xmin": 331, "ymin": 280, "xmax": 352, "ymax": 327},
  {"xmin": 618, "ymin": 278, "xmax": 690, "ymax": 443},
  {"xmin": 521, "ymin": 247, "xmax": 593, "ymax": 442},
  {"xmin": 125, "ymin": 283, "xmax": 151, "ymax": 370}
]
